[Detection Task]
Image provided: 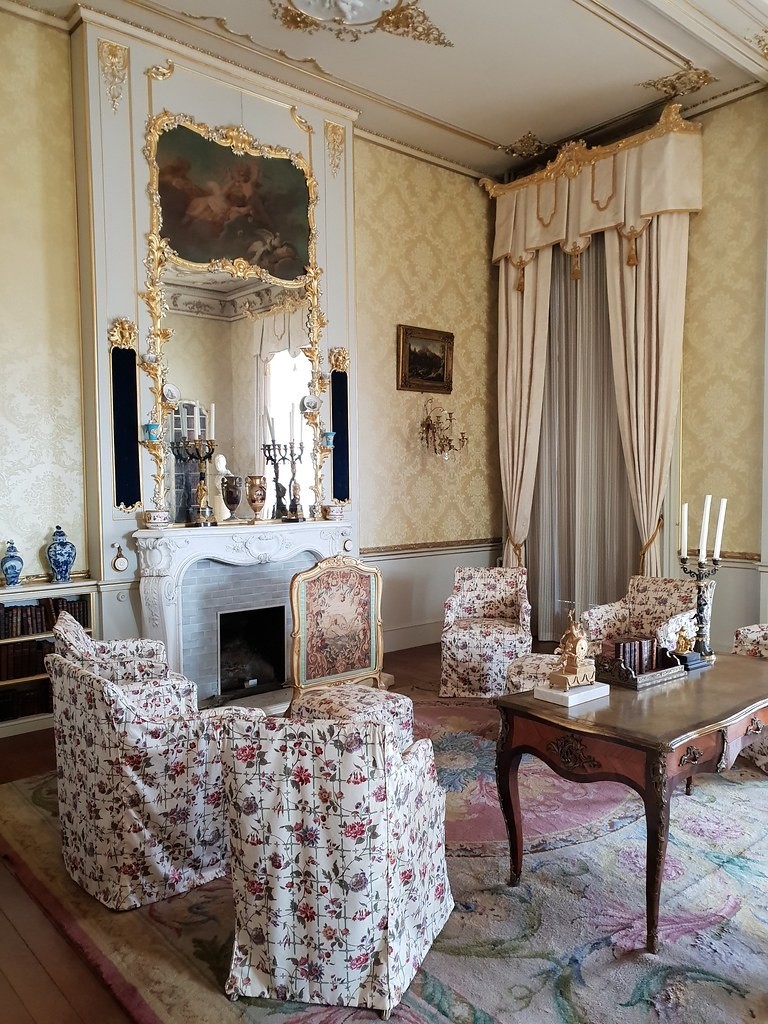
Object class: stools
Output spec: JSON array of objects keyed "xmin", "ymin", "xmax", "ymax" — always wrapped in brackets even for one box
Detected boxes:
[{"xmin": 499, "ymin": 652, "xmax": 596, "ymax": 735}]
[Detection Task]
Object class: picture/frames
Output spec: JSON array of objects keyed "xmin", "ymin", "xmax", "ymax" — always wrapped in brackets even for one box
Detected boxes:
[{"xmin": 395, "ymin": 324, "xmax": 455, "ymax": 396}]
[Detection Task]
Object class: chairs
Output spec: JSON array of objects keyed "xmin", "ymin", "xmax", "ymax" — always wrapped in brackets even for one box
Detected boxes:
[{"xmin": 0, "ymin": 565, "xmax": 768, "ymax": 1024}]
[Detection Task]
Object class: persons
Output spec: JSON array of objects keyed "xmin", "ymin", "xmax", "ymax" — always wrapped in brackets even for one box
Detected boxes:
[
  {"xmin": 551, "ymin": 643, "xmax": 575, "ymax": 674},
  {"xmin": 214, "ymin": 454, "xmax": 233, "ymax": 495},
  {"xmin": 675, "ymin": 626, "xmax": 695, "ymax": 651}
]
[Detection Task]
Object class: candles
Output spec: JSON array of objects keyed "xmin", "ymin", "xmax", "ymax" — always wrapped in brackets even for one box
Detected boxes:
[
  {"xmin": 290, "ymin": 412, "xmax": 292, "ymax": 441},
  {"xmin": 292, "ymin": 404, "xmax": 295, "ymax": 440},
  {"xmin": 699, "ymin": 495, "xmax": 713, "ymax": 559},
  {"xmin": 301, "ymin": 411, "xmax": 302, "ymax": 441},
  {"xmin": 208, "ymin": 402, "xmax": 215, "ymax": 440},
  {"xmin": 194, "ymin": 406, "xmax": 197, "ymax": 440},
  {"xmin": 714, "ymin": 498, "xmax": 727, "ymax": 556},
  {"xmin": 681, "ymin": 503, "xmax": 688, "ymax": 555},
  {"xmin": 169, "ymin": 405, "xmax": 183, "ymax": 444},
  {"xmin": 265, "ymin": 405, "xmax": 275, "ymax": 441},
  {"xmin": 197, "ymin": 400, "xmax": 200, "ymax": 438},
  {"xmin": 184, "ymin": 409, "xmax": 187, "ymax": 441}
]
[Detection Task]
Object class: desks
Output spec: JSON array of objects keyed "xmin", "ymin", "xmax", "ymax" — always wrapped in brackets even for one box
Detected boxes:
[{"xmin": 492, "ymin": 651, "xmax": 768, "ymax": 956}]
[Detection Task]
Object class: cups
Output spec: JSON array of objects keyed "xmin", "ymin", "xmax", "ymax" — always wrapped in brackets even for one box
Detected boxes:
[
  {"xmin": 145, "ymin": 509, "xmax": 170, "ymax": 529},
  {"xmin": 320, "ymin": 504, "xmax": 344, "ymax": 520}
]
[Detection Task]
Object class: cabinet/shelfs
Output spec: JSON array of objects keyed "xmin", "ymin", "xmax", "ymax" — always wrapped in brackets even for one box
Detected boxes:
[{"xmin": 0, "ymin": 587, "xmax": 96, "ymax": 737}]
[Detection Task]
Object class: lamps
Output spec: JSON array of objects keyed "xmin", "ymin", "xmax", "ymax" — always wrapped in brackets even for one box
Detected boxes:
[{"xmin": 415, "ymin": 398, "xmax": 468, "ymax": 457}]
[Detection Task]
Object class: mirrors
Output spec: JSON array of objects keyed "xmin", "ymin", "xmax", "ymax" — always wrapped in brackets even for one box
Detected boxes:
[{"xmin": 138, "ymin": 112, "xmax": 328, "ymax": 518}]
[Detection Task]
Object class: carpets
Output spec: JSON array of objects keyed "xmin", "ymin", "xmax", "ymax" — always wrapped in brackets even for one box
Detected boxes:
[{"xmin": 0, "ymin": 675, "xmax": 768, "ymax": 1024}]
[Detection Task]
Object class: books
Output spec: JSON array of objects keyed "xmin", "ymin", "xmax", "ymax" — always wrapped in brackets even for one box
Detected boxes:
[{"xmin": 602, "ymin": 636, "xmax": 657, "ymax": 676}]
